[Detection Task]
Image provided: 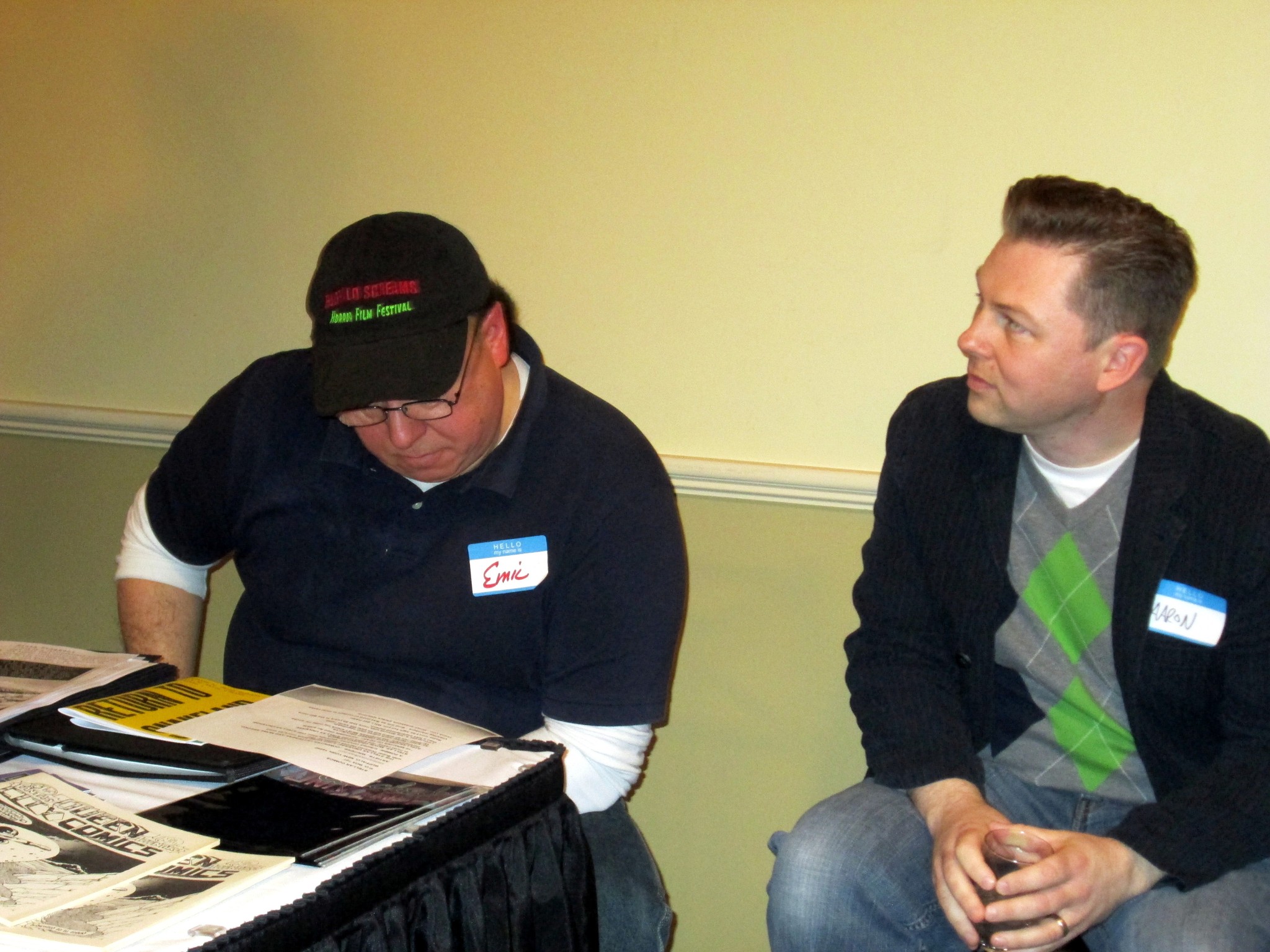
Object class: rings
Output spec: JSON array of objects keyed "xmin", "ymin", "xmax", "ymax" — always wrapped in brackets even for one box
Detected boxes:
[{"xmin": 1046, "ymin": 913, "xmax": 1069, "ymax": 939}]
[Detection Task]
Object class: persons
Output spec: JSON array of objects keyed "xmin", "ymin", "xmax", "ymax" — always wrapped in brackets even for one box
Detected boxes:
[
  {"xmin": 116, "ymin": 211, "xmax": 688, "ymax": 951},
  {"xmin": 765, "ymin": 174, "xmax": 1270, "ymax": 952}
]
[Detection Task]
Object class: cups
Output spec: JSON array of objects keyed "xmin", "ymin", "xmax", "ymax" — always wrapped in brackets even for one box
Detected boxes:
[{"xmin": 973, "ymin": 829, "xmax": 1054, "ymax": 952}]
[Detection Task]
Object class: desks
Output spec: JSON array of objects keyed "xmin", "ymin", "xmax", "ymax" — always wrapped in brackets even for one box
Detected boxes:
[{"xmin": 0, "ymin": 640, "xmax": 595, "ymax": 952}]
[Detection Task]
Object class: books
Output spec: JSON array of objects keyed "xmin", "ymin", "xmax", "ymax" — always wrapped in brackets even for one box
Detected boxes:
[{"xmin": 1, "ymin": 639, "xmax": 501, "ymax": 951}]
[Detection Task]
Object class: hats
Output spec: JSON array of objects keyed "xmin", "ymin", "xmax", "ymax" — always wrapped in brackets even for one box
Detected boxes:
[{"xmin": 306, "ymin": 212, "xmax": 490, "ymax": 416}]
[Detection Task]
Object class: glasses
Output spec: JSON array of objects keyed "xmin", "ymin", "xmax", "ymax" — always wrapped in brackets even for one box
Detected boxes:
[{"xmin": 336, "ymin": 314, "xmax": 482, "ymax": 427}]
